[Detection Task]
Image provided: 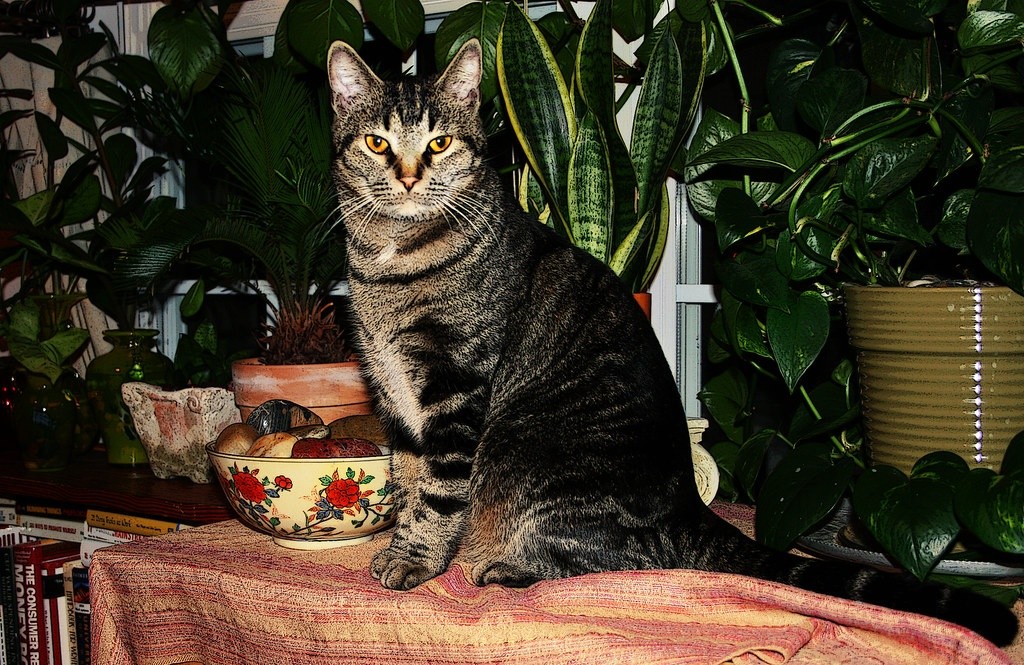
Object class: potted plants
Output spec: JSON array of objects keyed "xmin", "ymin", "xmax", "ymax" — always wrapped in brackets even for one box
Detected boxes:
[{"xmin": 0, "ymin": 0, "xmax": 1023, "ymax": 595}]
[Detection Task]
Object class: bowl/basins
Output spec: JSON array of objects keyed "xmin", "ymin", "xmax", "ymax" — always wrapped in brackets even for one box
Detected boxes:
[{"xmin": 203, "ymin": 439, "xmax": 399, "ymax": 550}]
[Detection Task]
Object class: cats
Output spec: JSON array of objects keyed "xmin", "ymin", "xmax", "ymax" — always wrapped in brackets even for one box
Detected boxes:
[{"xmin": 325, "ymin": 38, "xmax": 1017, "ymax": 651}]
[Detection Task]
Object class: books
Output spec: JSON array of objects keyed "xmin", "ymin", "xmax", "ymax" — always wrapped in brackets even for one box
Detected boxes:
[{"xmin": 1, "ymin": 497, "xmax": 196, "ymax": 665}]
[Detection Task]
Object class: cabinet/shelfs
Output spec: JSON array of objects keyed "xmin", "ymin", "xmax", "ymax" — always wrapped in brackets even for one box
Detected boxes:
[{"xmin": 0, "ymin": 444, "xmax": 238, "ymax": 525}]
